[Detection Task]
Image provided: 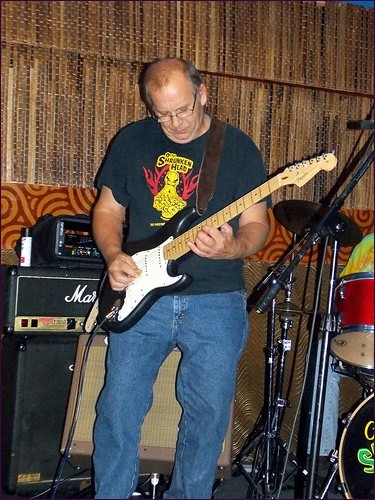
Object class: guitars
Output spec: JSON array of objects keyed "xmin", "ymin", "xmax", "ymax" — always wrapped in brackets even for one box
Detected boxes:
[{"xmin": 98, "ymin": 149, "xmax": 338, "ymax": 334}]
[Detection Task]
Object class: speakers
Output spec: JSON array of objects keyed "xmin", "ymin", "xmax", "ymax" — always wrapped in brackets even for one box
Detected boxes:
[
  {"xmin": 5, "ymin": 335, "xmax": 94, "ymax": 495},
  {"xmin": 59, "ymin": 333, "xmax": 232, "ymax": 480}
]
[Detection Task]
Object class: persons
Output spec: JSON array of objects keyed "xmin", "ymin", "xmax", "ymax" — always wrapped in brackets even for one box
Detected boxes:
[
  {"xmin": 307, "ymin": 231, "xmax": 375, "ymax": 480},
  {"xmin": 90, "ymin": 56, "xmax": 271, "ymax": 500}
]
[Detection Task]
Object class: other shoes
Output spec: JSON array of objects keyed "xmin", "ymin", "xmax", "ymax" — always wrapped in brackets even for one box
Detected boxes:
[{"xmin": 307, "ymin": 472, "xmax": 338, "ymax": 497}]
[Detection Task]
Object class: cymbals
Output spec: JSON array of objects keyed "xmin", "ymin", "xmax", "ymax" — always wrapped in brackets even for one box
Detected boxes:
[
  {"xmin": 247, "ymin": 302, "xmax": 336, "ymax": 316},
  {"xmin": 273, "ymin": 200, "xmax": 363, "ymax": 247}
]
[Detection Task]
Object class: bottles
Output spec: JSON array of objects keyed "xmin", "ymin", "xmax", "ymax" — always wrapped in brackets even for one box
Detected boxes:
[{"xmin": 19, "ymin": 227, "xmax": 33, "ymax": 267}]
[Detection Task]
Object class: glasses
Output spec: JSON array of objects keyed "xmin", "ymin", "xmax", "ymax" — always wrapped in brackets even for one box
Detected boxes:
[{"xmin": 149, "ymin": 89, "xmax": 198, "ymax": 123}]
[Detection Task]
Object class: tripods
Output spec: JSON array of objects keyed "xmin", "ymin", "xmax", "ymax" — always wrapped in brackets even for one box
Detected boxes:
[{"xmin": 209, "ymin": 121, "xmax": 375, "ymax": 499}]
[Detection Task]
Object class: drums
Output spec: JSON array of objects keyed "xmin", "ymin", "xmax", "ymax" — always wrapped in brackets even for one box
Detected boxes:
[
  {"xmin": 328, "ymin": 271, "xmax": 375, "ymax": 370},
  {"xmin": 339, "ymin": 393, "xmax": 375, "ymax": 499}
]
